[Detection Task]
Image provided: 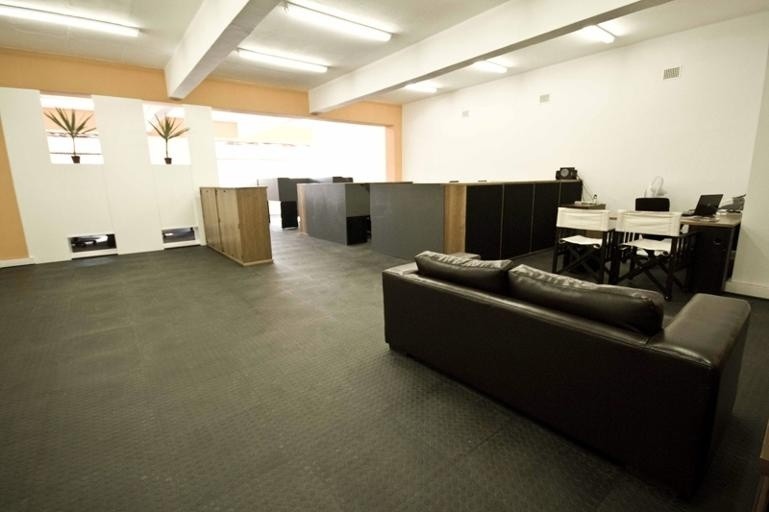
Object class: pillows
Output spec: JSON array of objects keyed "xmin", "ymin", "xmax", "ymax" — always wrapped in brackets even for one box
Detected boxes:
[
  {"xmin": 416, "ymin": 251, "xmax": 513, "ymax": 294},
  {"xmin": 509, "ymin": 263, "xmax": 667, "ymax": 336}
]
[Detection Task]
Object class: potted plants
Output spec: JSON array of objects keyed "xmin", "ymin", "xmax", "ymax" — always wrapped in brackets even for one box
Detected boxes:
[
  {"xmin": 149, "ymin": 113, "xmax": 189, "ymax": 165},
  {"xmin": 45, "ymin": 107, "xmax": 98, "ymax": 164}
]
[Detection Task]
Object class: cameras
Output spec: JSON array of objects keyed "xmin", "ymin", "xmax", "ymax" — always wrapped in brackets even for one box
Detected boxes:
[{"xmin": 555, "ymin": 167, "xmax": 577, "ymax": 179}]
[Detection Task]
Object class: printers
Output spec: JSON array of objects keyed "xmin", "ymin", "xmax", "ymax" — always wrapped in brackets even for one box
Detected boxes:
[{"xmin": 719, "ymin": 193, "xmax": 746, "ymax": 213}]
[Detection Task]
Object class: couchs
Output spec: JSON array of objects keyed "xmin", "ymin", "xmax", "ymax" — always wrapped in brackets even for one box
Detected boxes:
[{"xmin": 383, "ymin": 251, "xmax": 752, "ymax": 499}]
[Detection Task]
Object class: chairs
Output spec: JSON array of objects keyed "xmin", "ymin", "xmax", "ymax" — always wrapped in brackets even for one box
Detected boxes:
[
  {"xmin": 636, "ymin": 198, "xmax": 669, "ymax": 211},
  {"xmin": 615, "ymin": 212, "xmax": 696, "ymax": 302},
  {"xmin": 553, "ymin": 207, "xmax": 616, "ymax": 284}
]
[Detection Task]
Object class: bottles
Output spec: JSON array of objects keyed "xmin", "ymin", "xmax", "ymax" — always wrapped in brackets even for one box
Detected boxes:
[{"xmin": 594, "ymin": 194, "xmax": 597, "ymax": 204}]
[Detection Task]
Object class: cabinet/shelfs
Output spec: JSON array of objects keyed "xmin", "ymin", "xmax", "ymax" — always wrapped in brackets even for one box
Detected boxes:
[
  {"xmin": 465, "ymin": 179, "xmax": 582, "ymax": 259},
  {"xmin": 200, "ymin": 188, "xmax": 272, "ymax": 266}
]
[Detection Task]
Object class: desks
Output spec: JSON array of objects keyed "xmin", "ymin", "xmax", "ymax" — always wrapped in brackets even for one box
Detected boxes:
[{"xmin": 610, "ymin": 213, "xmax": 741, "ymax": 292}]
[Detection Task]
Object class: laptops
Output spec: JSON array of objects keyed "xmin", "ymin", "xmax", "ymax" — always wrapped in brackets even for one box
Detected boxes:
[{"xmin": 682, "ymin": 194, "xmax": 724, "ymax": 216}]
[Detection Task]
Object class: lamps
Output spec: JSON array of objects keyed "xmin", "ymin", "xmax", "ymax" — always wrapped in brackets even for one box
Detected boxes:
[
  {"xmin": 282, "ymin": 2, "xmax": 392, "ymax": 43},
  {"xmin": 585, "ymin": 24, "xmax": 615, "ymax": 44},
  {"xmin": 238, "ymin": 48, "xmax": 328, "ymax": 74},
  {"xmin": 1, "ymin": 5, "xmax": 140, "ymax": 36}
]
